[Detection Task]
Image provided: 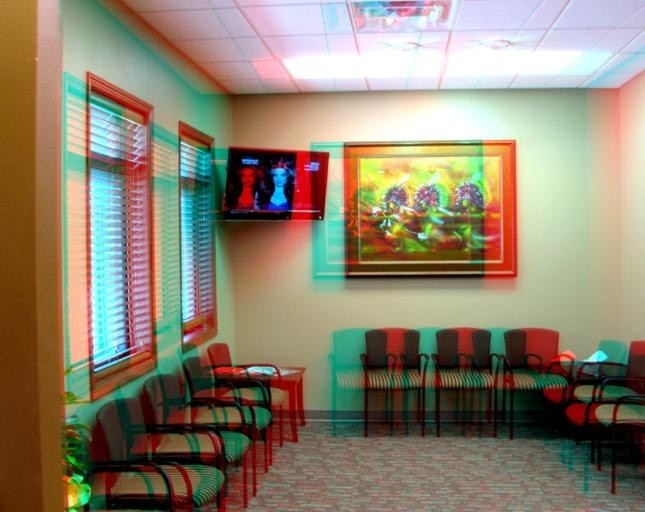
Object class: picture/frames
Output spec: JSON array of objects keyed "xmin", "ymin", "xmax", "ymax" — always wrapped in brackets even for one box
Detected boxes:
[{"xmin": 344, "ymin": 138, "xmax": 517, "ymax": 279}]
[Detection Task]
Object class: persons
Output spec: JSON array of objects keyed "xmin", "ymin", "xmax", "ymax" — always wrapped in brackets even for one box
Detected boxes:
[
  {"xmin": 255, "ymin": 164, "xmax": 297, "ymax": 211},
  {"xmin": 222, "ymin": 163, "xmax": 260, "ymax": 216}
]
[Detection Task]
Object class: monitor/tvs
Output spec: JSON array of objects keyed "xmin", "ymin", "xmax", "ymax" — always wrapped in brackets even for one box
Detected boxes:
[{"xmin": 221, "ymin": 145, "xmax": 330, "ymax": 221}]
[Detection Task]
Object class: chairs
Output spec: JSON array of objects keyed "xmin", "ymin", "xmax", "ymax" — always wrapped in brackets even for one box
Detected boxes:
[{"xmin": 83, "ymin": 340, "xmax": 285, "ymax": 512}]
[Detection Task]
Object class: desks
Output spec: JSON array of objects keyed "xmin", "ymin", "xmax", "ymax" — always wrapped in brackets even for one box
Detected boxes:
[{"xmin": 237, "ymin": 363, "xmax": 306, "ymax": 442}]
